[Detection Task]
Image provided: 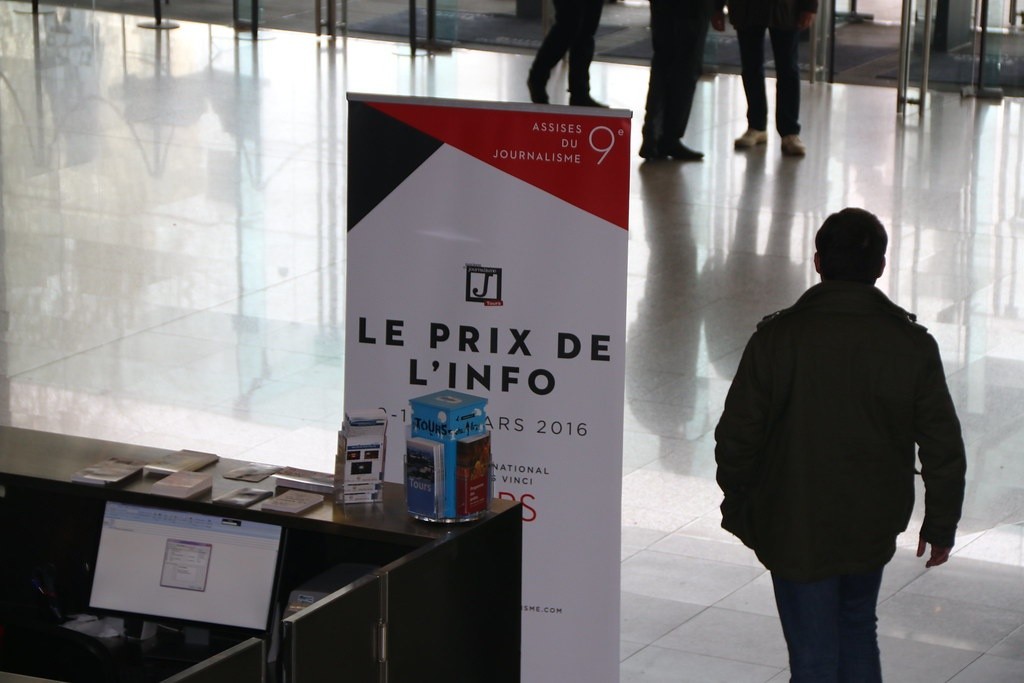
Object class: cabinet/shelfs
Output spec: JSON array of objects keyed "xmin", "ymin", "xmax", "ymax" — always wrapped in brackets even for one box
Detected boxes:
[{"xmin": 0, "ymin": 421, "xmax": 524, "ymax": 683}]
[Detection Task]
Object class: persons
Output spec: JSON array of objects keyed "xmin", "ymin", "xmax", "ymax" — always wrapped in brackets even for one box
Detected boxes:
[
  {"xmin": 639, "ymin": 0, "xmax": 718, "ymax": 163},
  {"xmin": 527, "ymin": 0, "xmax": 609, "ymax": 108},
  {"xmin": 714, "ymin": 206, "xmax": 966, "ymax": 683},
  {"xmin": 710, "ymin": 0, "xmax": 819, "ymax": 155}
]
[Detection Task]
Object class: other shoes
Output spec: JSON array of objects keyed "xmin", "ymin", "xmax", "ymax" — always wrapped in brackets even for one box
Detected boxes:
[
  {"xmin": 569, "ymin": 95, "xmax": 609, "ymax": 108},
  {"xmin": 639, "ymin": 142, "xmax": 668, "ymax": 159},
  {"xmin": 655, "ymin": 134, "xmax": 704, "ymax": 160},
  {"xmin": 527, "ymin": 79, "xmax": 550, "ymax": 104}
]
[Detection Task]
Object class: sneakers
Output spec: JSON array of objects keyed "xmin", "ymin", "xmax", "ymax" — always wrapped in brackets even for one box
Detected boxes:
[
  {"xmin": 734, "ymin": 127, "xmax": 767, "ymax": 147},
  {"xmin": 781, "ymin": 134, "xmax": 806, "ymax": 155}
]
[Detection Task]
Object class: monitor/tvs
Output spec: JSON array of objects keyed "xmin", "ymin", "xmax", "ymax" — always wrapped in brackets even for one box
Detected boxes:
[{"xmin": 85, "ymin": 497, "xmax": 290, "ymax": 663}]
